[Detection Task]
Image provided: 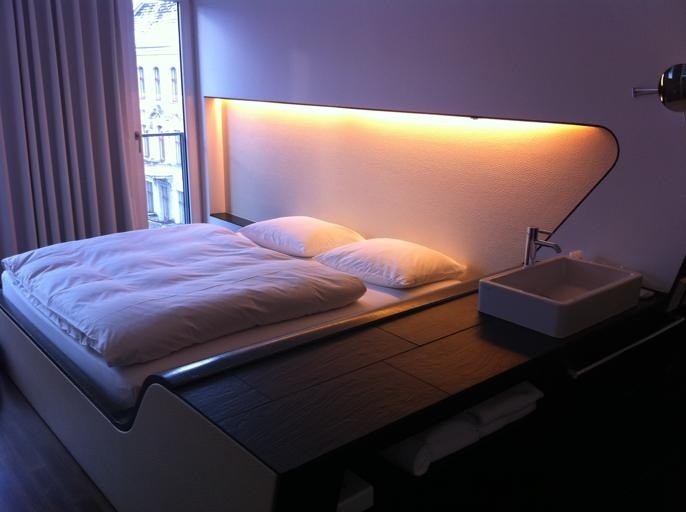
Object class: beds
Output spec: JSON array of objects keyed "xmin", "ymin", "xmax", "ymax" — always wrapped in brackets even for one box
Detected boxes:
[{"xmin": 0, "ymin": 97, "xmax": 619, "ymax": 511}]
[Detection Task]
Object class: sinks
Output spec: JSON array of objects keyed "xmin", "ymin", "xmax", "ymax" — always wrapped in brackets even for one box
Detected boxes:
[{"xmin": 478, "ymin": 255, "xmax": 644, "ymax": 340}]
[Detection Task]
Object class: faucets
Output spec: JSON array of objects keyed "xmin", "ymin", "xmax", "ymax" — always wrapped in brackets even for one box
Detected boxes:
[{"xmin": 523, "ymin": 226, "xmax": 562, "ymax": 267}]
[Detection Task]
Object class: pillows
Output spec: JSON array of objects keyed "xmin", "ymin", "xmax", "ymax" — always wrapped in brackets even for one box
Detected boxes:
[
  {"xmin": 237, "ymin": 212, "xmax": 366, "ymax": 258},
  {"xmin": 313, "ymin": 237, "xmax": 467, "ymax": 289}
]
